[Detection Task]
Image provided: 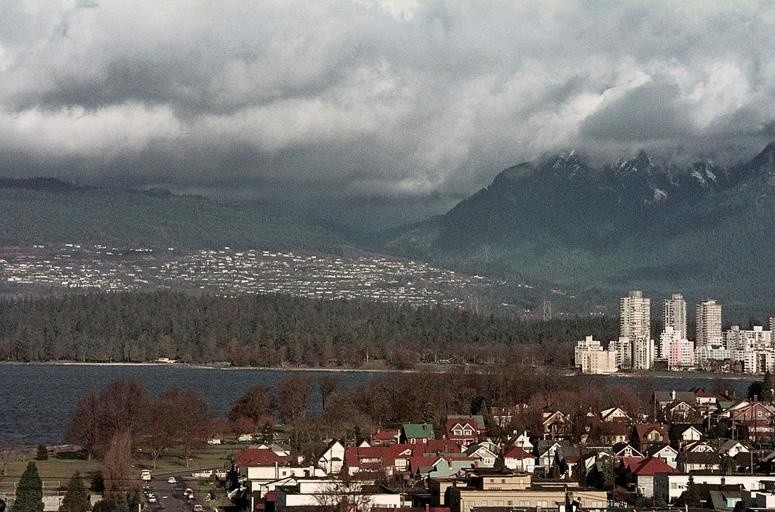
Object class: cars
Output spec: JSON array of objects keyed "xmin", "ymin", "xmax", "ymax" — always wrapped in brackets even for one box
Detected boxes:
[{"xmin": 141, "ymin": 469, "xmax": 206, "ymax": 512}]
